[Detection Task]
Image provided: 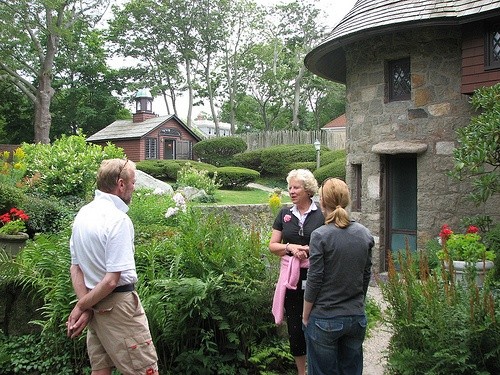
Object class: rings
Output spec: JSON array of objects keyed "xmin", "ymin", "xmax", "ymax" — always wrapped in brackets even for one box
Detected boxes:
[
  {"xmin": 297, "ymin": 253, "xmax": 299, "ymax": 254},
  {"xmin": 305, "ymin": 245, "xmax": 309, "ymax": 248}
]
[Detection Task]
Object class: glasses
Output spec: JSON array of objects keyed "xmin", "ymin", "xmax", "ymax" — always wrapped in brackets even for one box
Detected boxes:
[
  {"xmin": 118, "ymin": 158, "xmax": 129, "ymax": 177},
  {"xmin": 321, "ymin": 176, "xmax": 347, "ymax": 208},
  {"xmin": 298, "ymin": 219, "xmax": 305, "ymax": 236}
]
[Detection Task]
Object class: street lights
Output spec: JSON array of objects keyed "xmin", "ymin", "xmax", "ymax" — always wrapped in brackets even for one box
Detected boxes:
[{"xmin": 314, "ymin": 138, "xmax": 321, "ymax": 169}]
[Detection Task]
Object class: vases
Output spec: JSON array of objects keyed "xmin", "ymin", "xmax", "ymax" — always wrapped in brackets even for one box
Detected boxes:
[
  {"xmin": 450, "ymin": 259, "xmax": 496, "ymax": 301},
  {"xmin": 0, "ymin": 231, "xmax": 27, "ymax": 261}
]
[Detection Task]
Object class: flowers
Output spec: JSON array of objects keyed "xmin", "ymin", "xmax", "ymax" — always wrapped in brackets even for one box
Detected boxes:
[
  {"xmin": 437, "ymin": 222, "xmax": 494, "ymax": 263},
  {"xmin": 284, "ymin": 214, "xmax": 291, "ymax": 223},
  {"xmin": 0, "ymin": 208, "xmax": 28, "ymax": 236}
]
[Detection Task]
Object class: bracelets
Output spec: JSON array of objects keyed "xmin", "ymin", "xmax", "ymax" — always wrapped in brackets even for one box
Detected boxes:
[{"xmin": 284, "ymin": 242, "xmax": 289, "ymax": 254}]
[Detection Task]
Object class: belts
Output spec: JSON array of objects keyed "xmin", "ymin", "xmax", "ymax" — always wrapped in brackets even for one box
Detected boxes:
[{"xmin": 113, "ymin": 283, "xmax": 135, "ymax": 292}]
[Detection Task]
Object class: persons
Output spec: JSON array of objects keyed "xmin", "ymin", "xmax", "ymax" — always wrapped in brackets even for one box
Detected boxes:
[
  {"xmin": 302, "ymin": 176, "xmax": 375, "ymax": 375},
  {"xmin": 269, "ymin": 169, "xmax": 326, "ymax": 375},
  {"xmin": 66, "ymin": 158, "xmax": 159, "ymax": 375}
]
